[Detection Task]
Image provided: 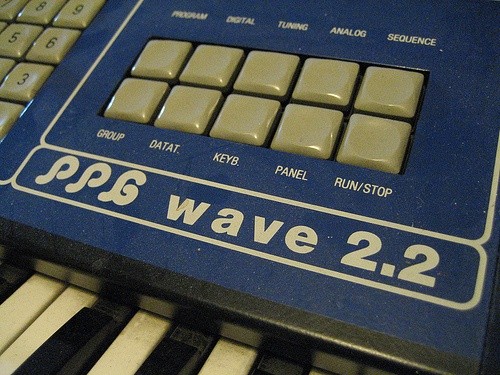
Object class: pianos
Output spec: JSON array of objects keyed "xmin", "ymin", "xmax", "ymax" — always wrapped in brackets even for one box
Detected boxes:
[{"xmin": 0, "ymin": 248, "xmax": 339, "ymax": 375}]
